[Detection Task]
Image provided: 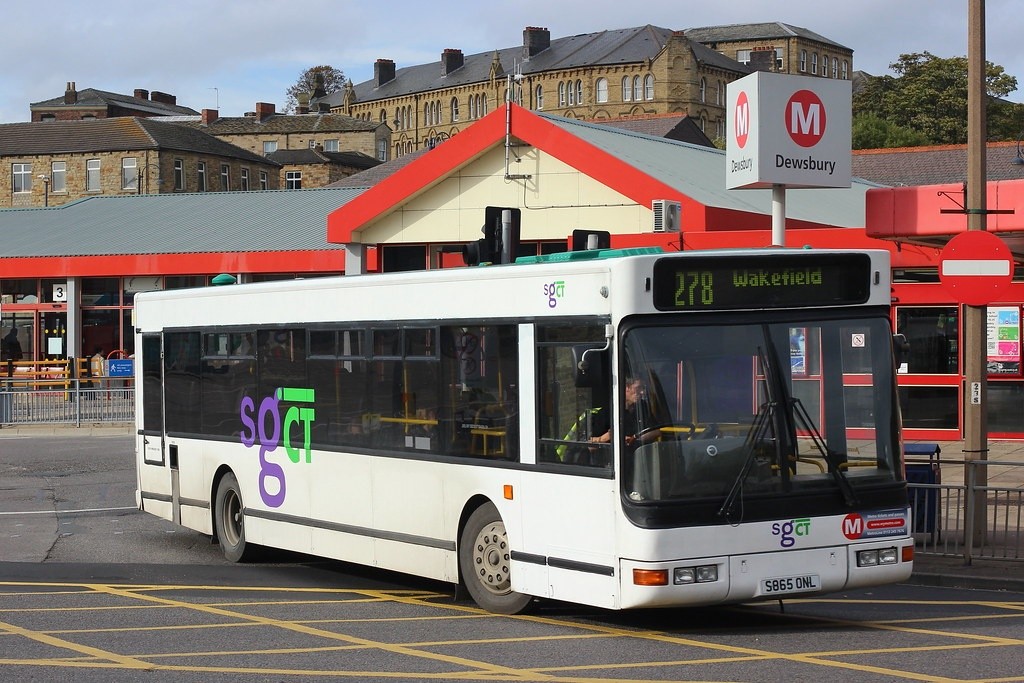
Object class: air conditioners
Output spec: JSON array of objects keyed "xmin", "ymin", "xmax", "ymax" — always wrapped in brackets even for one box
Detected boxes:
[{"xmin": 652, "ymin": 200, "xmax": 682, "ymax": 234}]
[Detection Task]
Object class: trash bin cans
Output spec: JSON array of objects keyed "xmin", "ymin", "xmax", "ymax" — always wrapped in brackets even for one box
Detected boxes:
[{"xmin": 902, "ymin": 443, "xmax": 942, "ymax": 544}]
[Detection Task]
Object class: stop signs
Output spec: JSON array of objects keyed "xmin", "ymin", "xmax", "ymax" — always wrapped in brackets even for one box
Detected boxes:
[{"xmin": 937, "ymin": 231, "xmax": 1014, "ymax": 306}]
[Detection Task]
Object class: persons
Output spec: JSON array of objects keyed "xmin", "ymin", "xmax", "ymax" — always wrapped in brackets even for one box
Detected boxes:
[
  {"xmin": 3, "ymin": 328, "xmax": 23, "ymax": 372},
  {"xmin": 583, "ymin": 372, "xmax": 659, "ymax": 465},
  {"xmin": 91, "ymin": 349, "xmax": 105, "ymax": 399}
]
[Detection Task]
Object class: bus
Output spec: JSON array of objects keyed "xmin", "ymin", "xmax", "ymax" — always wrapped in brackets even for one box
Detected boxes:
[{"xmin": 133, "ymin": 245, "xmax": 915, "ymax": 615}]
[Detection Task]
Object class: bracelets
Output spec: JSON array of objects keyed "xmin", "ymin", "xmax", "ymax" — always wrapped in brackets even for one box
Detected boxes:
[{"xmin": 598, "ymin": 436, "xmax": 602, "ymax": 443}]
